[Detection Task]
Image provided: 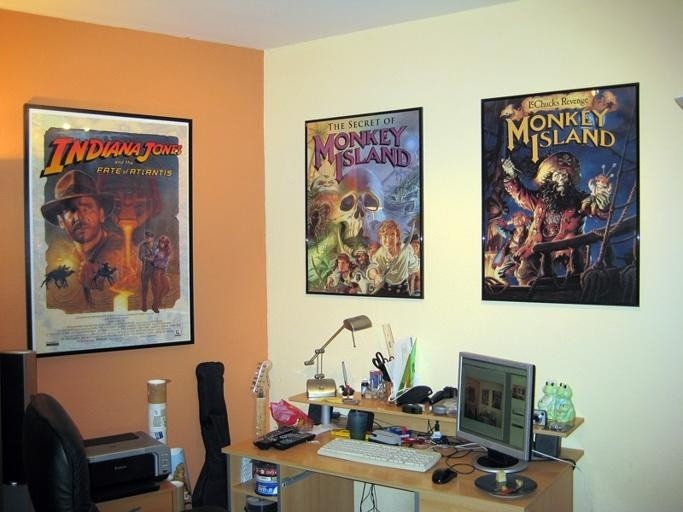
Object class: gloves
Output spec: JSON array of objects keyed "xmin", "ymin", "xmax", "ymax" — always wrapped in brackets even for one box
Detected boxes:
[
  {"xmin": 502, "ymin": 156, "xmax": 514, "ymax": 177},
  {"xmin": 595, "ymin": 191, "xmax": 611, "ymax": 212}
]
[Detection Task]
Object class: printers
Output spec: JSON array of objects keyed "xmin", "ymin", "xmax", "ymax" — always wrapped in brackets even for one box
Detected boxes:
[{"xmin": 81, "ymin": 430, "xmax": 173, "ymax": 503}]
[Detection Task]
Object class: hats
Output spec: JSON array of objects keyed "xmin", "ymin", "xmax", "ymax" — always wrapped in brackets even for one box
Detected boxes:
[
  {"xmin": 531, "ymin": 150, "xmax": 580, "ymax": 187},
  {"xmin": 41, "ymin": 168, "xmax": 115, "ymax": 228}
]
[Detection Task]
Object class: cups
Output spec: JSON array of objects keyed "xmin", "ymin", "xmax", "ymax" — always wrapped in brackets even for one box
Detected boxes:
[
  {"xmin": 349, "ymin": 410, "xmax": 368, "ymax": 440},
  {"xmin": 171, "ymin": 447, "xmax": 193, "ymax": 503}
]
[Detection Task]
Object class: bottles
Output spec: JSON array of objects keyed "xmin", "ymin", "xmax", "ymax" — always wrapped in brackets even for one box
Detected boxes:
[
  {"xmin": 146, "ymin": 379, "xmax": 168, "ymax": 447},
  {"xmin": 359, "ymin": 370, "xmax": 391, "ymax": 400},
  {"xmin": 432, "ymin": 420, "xmax": 441, "ymax": 439},
  {"xmin": 401, "ymin": 426, "xmax": 406, "ymax": 434}
]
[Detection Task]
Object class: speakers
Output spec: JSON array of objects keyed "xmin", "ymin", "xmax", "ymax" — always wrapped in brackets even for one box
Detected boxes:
[
  {"xmin": 308, "ymin": 404, "xmax": 333, "ymax": 425},
  {"xmin": 536, "ymin": 435, "xmax": 561, "ymax": 460},
  {"xmin": 346, "ymin": 410, "xmax": 375, "ymax": 440}
]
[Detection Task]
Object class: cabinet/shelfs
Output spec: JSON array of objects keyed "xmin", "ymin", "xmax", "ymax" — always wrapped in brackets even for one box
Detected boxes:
[{"xmin": 93, "ymin": 480, "xmax": 186, "ymax": 511}]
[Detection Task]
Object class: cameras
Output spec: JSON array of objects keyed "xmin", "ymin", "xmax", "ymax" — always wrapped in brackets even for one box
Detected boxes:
[{"xmin": 533, "ymin": 409, "xmax": 547, "ymax": 426}]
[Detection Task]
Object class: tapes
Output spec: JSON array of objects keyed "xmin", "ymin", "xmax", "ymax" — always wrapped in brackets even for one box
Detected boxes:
[{"xmin": 403, "ymin": 404, "xmax": 422, "ymax": 415}]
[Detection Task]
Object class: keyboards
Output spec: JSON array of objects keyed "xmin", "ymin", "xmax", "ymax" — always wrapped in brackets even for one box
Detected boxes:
[{"xmin": 317, "ymin": 437, "xmax": 443, "ymax": 473}]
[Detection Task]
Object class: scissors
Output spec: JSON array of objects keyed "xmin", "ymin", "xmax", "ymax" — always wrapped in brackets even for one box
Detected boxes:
[{"xmin": 373, "ymin": 352, "xmax": 395, "ymax": 382}]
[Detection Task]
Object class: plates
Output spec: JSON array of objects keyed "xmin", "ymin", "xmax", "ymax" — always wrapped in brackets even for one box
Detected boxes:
[{"xmin": 474, "ymin": 472, "xmax": 537, "ymax": 500}]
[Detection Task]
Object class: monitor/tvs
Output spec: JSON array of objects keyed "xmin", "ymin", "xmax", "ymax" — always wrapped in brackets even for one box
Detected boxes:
[{"xmin": 455, "ymin": 351, "xmax": 536, "ymax": 475}]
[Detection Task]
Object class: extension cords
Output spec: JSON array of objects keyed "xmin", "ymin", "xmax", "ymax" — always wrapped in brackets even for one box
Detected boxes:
[{"xmin": 431, "ymin": 429, "xmax": 441, "ymax": 439}]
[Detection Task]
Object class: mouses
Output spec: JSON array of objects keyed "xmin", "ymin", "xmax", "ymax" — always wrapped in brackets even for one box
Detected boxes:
[{"xmin": 431, "ymin": 465, "xmax": 458, "ymax": 485}]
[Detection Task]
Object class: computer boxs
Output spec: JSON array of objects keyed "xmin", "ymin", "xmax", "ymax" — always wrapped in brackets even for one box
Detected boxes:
[{"xmin": 353, "ymin": 481, "xmax": 417, "ymax": 511}]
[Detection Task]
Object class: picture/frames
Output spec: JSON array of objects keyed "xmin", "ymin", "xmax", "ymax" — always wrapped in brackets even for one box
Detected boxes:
[
  {"xmin": 303, "ymin": 105, "xmax": 424, "ymax": 301},
  {"xmin": 479, "ymin": 81, "xmax": 641, "ymax": 309},
  {"xmin": 22, "ymin": 102, "xmax": 196, "ymax": 358}
]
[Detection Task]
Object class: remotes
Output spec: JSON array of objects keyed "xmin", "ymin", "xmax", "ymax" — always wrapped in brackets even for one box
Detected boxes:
[
  {"xmin": 273, "ymin": 432, "xmax": 316, "ymax": 449},
  {"xmin": 251, "ymin": 426, "xmax": 294, "ymax": 440},
  {"xmin": 255, "ymin": 432, "xmax": 296, "ymax": 449}
]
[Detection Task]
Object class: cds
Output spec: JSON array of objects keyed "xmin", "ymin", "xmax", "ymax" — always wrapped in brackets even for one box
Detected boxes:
[{"xmin": 244, "ymin": 496, "xmax": 277, "ymax": 512}]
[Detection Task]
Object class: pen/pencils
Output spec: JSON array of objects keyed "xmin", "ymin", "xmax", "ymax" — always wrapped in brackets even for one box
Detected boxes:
[{"xmin": 342, "ymin": 361, "xmax": 347, "ymax": 384}]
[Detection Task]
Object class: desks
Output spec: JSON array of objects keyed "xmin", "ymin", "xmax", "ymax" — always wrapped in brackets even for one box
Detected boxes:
[{"xmin": 220, "ymin": 392, "xmax": 585, "ymax": 512}]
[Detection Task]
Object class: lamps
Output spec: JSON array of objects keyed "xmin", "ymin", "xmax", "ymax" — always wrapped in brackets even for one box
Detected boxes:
[{"xmin": 303, "ymin": 313, "xmax": 372, "ymax": 398}]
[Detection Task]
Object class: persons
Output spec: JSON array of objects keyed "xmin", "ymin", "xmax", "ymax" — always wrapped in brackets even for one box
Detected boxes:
[
  {"xmin": 307, "ymin": 220, "xmax": 421, "ymax": 296},
  {"xmin": 492, "ymin": 152, "xmax": 613, "ymax": 285},
  {"xmin": 139, "ymin": 231, "xmax": 159, "ymax": 313},
  {"xmin": 151, "ymin": 235, "xmax": 172, "ymax": 314},
  {"xmin": 40, "ymin": 169, "xmax": 123, "ymax": 307}
]
